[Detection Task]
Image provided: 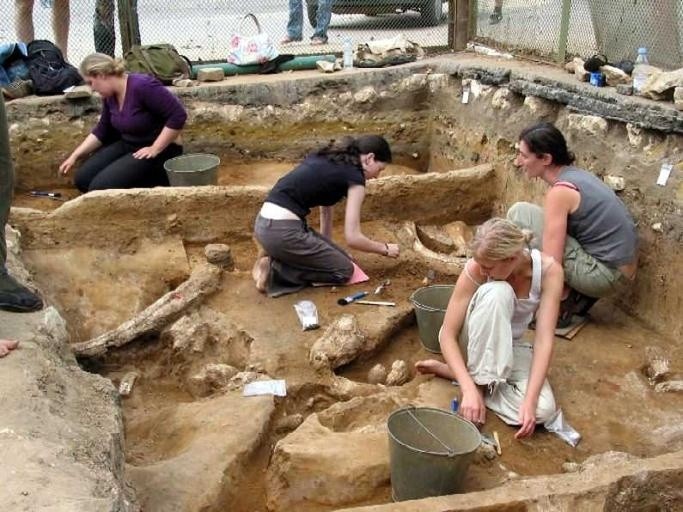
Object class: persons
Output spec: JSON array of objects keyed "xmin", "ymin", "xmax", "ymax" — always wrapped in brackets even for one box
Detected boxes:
[
  {"xmin": 14, "ymin": 0, "xmax": 70, "ymax": 64},
  {"xmin": 0, "ymin": 338, "xmax": 19, "ymax": 358},
  {"xmin": 58, "ymin": 53, "xmax": 187, "ymax": 195},
  {"xmin": 0, "ymin": 82, "xmax": 43, "ymax": 313},
  {"xmin": 505, "ymin": 121, "xmax": 638, "ymax": 329},
  {"xmin": 414, "ymin": 218, "xmax": 565, "ymax": 440},
  {"xmin": 252, "ymin": 133, "xmax": 400, "ymax": 298},
  {"xmin": 278, "ymin": 0, "xmax": 333, "ymax": 46},
  {"xmin": 90, "ymin": 1, "xmax": 144, "ymax": 59}
]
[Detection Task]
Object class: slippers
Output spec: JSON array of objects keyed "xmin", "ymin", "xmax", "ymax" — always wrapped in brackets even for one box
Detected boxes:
[{"xmin": 584, "ymin": 55, "xmax": 607, "ymax": 73}]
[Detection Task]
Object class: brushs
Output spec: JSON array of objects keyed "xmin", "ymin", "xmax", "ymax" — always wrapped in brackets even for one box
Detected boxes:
[
  {"xmin": 31, "ymin": 192, "xmax": 61, "ymax": 197},
  {"xmin": 338, "ymin": 291, "xmax": 369, "ymax": 305}
]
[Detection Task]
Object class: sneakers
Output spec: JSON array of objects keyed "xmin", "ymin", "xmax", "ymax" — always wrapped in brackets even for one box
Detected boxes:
[
  {"xmin": 1, "ymin": 77, "xmax": 32, "ymax": 98},
  {"xmin": 279, "ymin": 36, "xmax": 302, "ymax": 44},
  {"xmin": 573, "ymin": 296, "xmax": 600, "ymax": 316},
  {"xmin": 0, "ymin": 274, "xmax": 43, "ymax": 311},
  {"xmin": 312, "ymin": 36, "xmax": 327, "ymax": 45},
  {"xmin": 488, "ymin": 12, "xmax": 502, "ymax": 24},
  {"xmin": 556, "ymin": 289, "xmax": 581, "ymax": 328}
]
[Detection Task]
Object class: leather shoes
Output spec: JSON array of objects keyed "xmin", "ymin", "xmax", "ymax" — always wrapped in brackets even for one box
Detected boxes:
[{"xmin": 259, "ymin": 54, "xmax": 294, "ymax": 73}]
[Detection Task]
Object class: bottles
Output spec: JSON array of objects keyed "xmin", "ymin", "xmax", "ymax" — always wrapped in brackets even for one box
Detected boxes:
[
  {"xmin": 487, "ymin": 51, "xmax": 514, "ymax": 59},
  {"xmin": 343, "ymin": 38, "xmax": 353, "ymax": 70},
  {"xmin": 467, "ymin": 40, "xmax": 497, "ymax": 56},
  {"xmin": 632, "ymin": 47, "xmax": 649, "ymax": 96}
]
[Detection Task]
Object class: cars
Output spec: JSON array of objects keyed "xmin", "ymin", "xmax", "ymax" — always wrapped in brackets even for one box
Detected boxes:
[{"xmin": 306, "ymin": 0, "xmax": 448, "ymax": 30}]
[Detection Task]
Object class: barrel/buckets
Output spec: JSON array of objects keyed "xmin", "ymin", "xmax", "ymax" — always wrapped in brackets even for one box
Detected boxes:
[
  {"xmin": 410, "ymin": 285, "xmax": 453, "ymax": 354},
  {"xmin": 164, "ymin": 154, "xmax": 221, "ymax": 186},
  {"xmin": 386, "ymin": 393, "xmax": 483, "ymax": 501}
]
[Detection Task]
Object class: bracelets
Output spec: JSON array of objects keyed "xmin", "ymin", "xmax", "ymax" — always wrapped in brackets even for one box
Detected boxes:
[{"xmin": 382, "ymin": 243, "xmax": 389, "ymax": 256}]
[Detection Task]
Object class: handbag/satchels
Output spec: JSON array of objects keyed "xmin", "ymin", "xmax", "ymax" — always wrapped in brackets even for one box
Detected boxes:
[
  {"xmin": 227, "ymin": 13, "xmax": 278, "ymax": 65},
  {"xmin": 27, "ymin": 41, "xmax": 83, "ymax": 96},
  {"xmin": 353, "ymin": 33, "xmax": 425, "ymax": 67},
  {"xmin": 123, "ymin": 44, "xmax": 193, "ymax": 85}
]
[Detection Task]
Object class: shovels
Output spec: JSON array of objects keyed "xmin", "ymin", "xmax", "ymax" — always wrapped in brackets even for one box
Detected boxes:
[
  {"xmin": 476, "ymin": 419, "xmax": 498, "ymax": 447},
  {"xmin": 422, "ymin": 270, "xmax": 435, "ymax": 285}
]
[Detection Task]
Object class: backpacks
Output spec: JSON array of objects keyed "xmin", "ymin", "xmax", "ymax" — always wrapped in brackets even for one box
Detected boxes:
[{"xmin": 0, "ymin": 42, "xmax": 29, "ymax": 83}]
